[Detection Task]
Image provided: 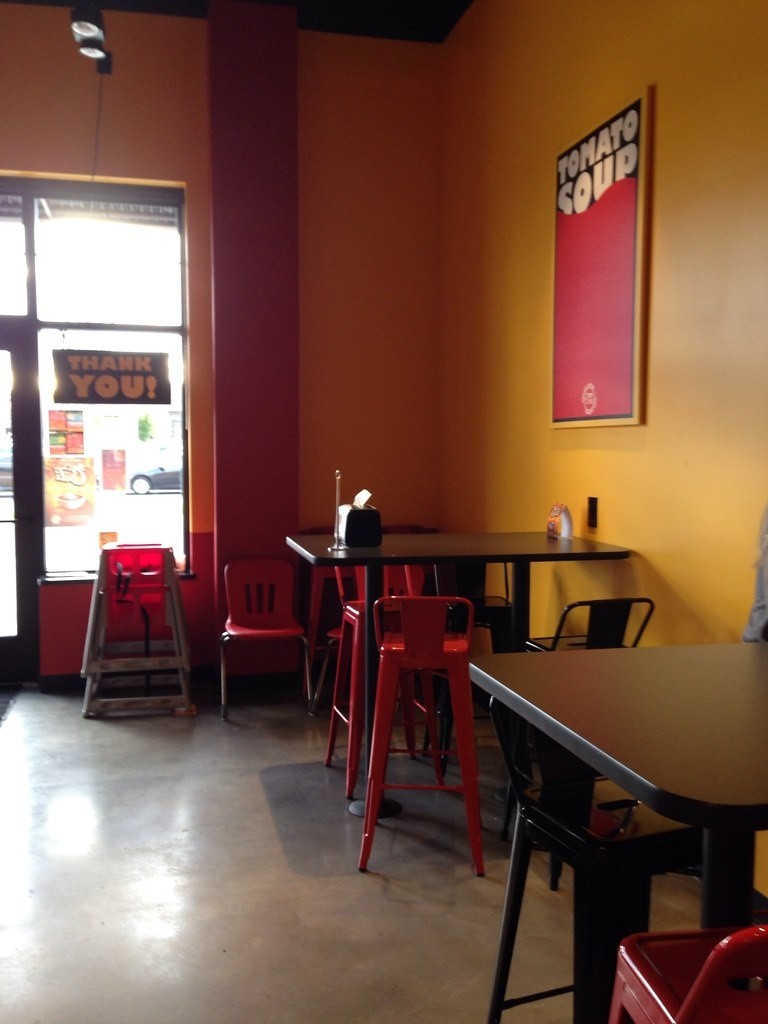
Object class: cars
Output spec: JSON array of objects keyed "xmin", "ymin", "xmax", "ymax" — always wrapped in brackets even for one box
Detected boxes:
[{"xmin": 130, "ymin": 465, "xmax": 183, "ymax": 494}]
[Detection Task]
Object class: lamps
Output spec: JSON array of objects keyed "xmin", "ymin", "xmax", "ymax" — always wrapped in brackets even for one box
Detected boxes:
[{"xmin": 72, "ymin": 1, "xmax": 107, "ymax": 61}]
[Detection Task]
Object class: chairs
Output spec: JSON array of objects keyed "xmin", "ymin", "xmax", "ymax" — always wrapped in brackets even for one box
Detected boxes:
[
  {"xmin": 359, "ymin": 595, "xmax": 486, "ymax": 878},
  {"xmin": 221, "ymin": 560, "xmax": 315, "ymax": 721},
  {"xmin": 486, "ymin": 695, "xmax": 704, "ymax": 1024},
  {"xmin": 607, "ymin": 926, "xmax": 767, "ymax": 1024},
  {"xmin": 298, "ymin": 547, "xmax": 441, "ymax": 718},
  {"xmin": 323, "ymin": 561, "xmax": 445, "ymax": 796},
  {"xmin": 418, "ymin": 560, "xmax": 515, "ymax": 776},
  {"xmin": 500, "ymin": 596, "xmax": 657, "ymax": 890}
]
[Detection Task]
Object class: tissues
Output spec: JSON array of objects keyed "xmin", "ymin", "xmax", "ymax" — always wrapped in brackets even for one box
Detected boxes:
[{"xmin": 333, "ymin": 489, "xmax": 383, "ymax": 548}]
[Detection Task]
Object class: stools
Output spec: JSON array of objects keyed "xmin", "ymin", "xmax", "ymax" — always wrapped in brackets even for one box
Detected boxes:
[{"xmin": 80, "ymin": 543, "xmax": 198, "ymax": 727}]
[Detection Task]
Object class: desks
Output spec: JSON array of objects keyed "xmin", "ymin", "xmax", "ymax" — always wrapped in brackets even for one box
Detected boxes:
[
  {"xmin": 286, "ymin": 533, "xmax": 631, "ymax": 819},
  {"xmin": 467, "ymin": 643, "xmax": 768, "ymax": 996}
]
